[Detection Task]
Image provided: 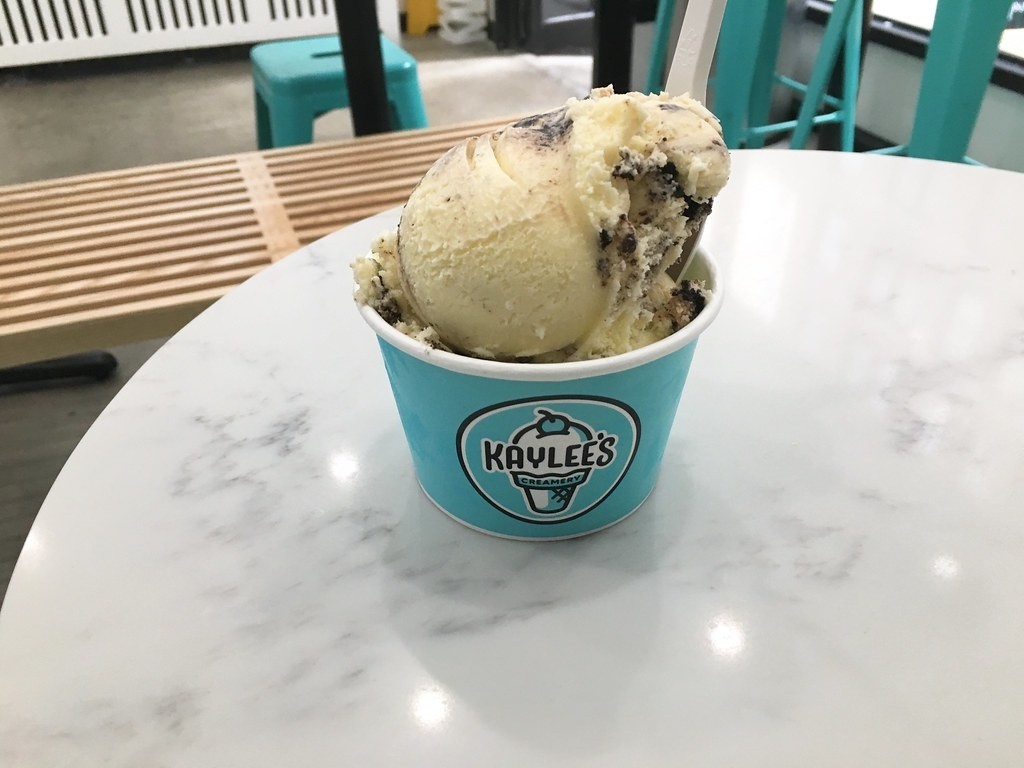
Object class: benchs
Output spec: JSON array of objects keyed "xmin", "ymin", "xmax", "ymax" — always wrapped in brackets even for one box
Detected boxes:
[{"xmin": 0, "ymin": 109, "xmax": 541, "ymax": 370}]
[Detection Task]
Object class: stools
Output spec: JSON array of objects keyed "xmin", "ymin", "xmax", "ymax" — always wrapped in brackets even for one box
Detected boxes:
[{"xmin": 249, "ymin": 36, "xmax": 426, "ymax": 151}]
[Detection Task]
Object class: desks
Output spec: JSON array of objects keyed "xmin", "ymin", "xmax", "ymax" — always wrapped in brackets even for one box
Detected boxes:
[{"xmin": 0, "ymin": 147, "xmax": 1024, "ymax": 768}]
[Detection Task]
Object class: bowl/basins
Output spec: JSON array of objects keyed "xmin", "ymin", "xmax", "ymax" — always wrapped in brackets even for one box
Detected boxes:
[{"xmin": 354, "ymin": 246, "xmax": 726, "ymax": 543}]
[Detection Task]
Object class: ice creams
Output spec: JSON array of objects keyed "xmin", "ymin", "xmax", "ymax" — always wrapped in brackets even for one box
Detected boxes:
[{"xmin": 350, "ymin": 83, "xmax": 731, "ymax": 543}]
